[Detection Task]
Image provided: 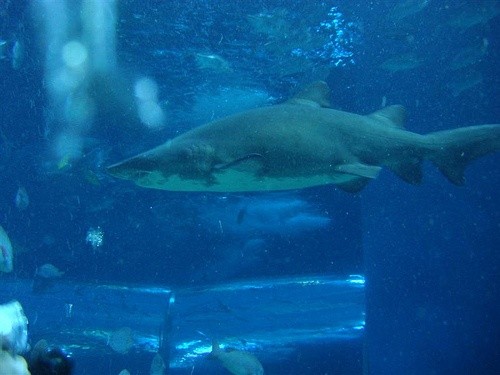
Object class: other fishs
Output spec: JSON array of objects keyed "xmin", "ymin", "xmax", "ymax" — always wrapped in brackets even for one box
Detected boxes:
[
  {"xmin": 1, "ymin": 209, "xmax": 356, "ymax": 375},
  {"xmin": 3, "ymin": 0, "xmax": 500, "ymax": 113},
  {"xmin": 1, "ymin": 130, "xmax": 121, "ymax": 210}
]
